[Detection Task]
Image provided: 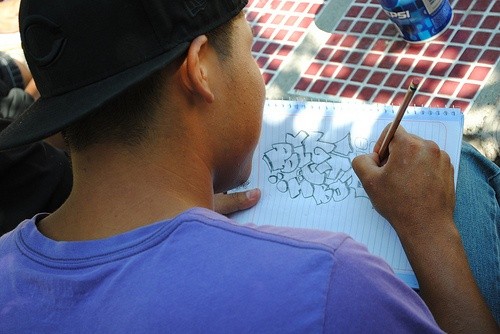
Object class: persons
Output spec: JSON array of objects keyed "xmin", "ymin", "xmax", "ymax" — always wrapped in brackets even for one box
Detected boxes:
[
  {"xmin": 0, "ymin": 0, "xmax": 500, "ymax": 334},
  {"xmin": 0, "ymin": 52, "xmax": 74, "ymax": 224}
]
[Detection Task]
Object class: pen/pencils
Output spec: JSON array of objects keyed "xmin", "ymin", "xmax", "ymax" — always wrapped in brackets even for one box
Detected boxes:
[{"xmin": 376, "ymin": 78, "xmax": 422, "ymax": 161}]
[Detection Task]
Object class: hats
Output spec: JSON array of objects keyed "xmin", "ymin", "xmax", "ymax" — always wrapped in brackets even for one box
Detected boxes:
[{"xmin": 0, "ymin": 0, "xmax": 248, "ymax": 153}]
